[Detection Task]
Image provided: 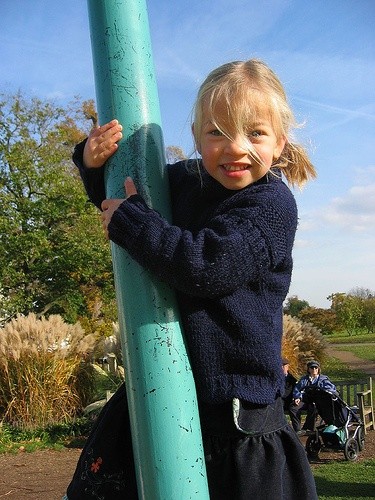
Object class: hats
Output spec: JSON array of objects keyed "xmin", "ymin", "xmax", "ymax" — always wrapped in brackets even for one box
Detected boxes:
[{"xmin": 307, "ymin": 361, "xmax": 320, "ymax": 367}]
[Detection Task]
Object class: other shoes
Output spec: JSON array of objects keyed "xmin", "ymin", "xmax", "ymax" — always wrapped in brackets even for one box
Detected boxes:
[{"xmin": 296, "ymin": 429, "xmax": 306, "ymax": 436}]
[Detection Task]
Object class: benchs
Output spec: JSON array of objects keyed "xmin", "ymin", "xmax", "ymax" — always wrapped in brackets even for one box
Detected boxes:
[{"xmin": 284, "ymin": 377, "xmax": 375, "ymax": 433}]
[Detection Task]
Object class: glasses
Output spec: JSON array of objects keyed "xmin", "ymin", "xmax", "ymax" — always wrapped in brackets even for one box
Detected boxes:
[{"xmin": 309, "ymin": 366, "xmax": 318, "ymax": 369}]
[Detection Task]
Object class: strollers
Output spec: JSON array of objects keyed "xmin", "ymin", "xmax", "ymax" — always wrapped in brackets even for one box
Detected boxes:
[{"xmin": 306, "ymin": 387, "xmax": 364, "ymax": 461}]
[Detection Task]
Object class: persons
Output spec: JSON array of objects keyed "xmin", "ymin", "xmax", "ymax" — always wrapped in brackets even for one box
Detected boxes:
[
  {"xmin": 290, "ymin": 360, "xmax": 336, "ymax": 436},
  {"xmin": 282, "ymin": 358, "xmax": 318, "ymax": 433},
  {"xmin": 66, "ymin": 60, "xmax": 318, "ymax": 500}
]
